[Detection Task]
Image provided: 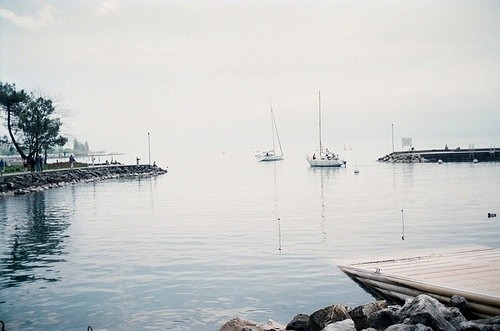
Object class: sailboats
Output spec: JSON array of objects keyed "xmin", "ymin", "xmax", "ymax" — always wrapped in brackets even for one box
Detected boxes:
[
  {"xmin": 305, "ymin": 90, "xmax": 344, "ymax": 167},
  {"xmin": 255, "ymin": 94, "xmax": 285, "ymax": 163}
]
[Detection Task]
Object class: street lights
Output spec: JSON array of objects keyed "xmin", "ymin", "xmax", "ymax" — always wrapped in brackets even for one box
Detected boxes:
[
  {"xmin": 148, "ymin": 132, "xmax": 151, "ymax": 165},
  {"xmin": 392, "ymin": 123, "xmax": 395, "ymax": 153}
]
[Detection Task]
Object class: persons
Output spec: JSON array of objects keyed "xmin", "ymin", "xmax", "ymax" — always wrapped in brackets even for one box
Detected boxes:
[
  {"xmin": 313, "ymin": 153, "xmax": 316, "ymax": 159},
  {"xmin": 70, "ymin": 155, "xmax": 75, "ymax": 168},
  {"xmin": 30, "ymin": 154, "xmax": 43, "ymax": 172},
  {"xmin": 91, "ymin": 155, "xmax": 95, "ymax": 166},
  {"xmin": 0, "ymin": 157, "xmax": 5, "ymax": 175}
]
